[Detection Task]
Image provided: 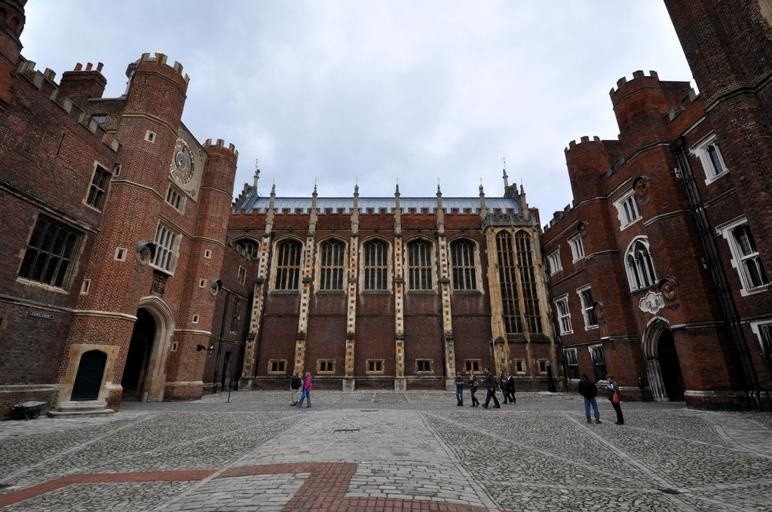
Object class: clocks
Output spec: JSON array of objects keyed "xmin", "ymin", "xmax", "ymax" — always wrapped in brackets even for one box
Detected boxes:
[{"xmin": 170, "ymin": 136, "xmax": 195, "ymax": 183}]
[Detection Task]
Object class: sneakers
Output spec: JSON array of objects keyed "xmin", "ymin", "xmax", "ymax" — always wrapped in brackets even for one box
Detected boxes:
[
  {"xmin": 457, "ymin": 398, "xmax": 516, "ymax": 409},
  {"xmin": 290, "ymin": 402, "xmax": 311, "ymax": 408},
  {"xmin": 614, "ymin": 420, "xmax": 624, "ymax": 425},
  {"xmin": 587, "ymin": 420, "xmax": 601, "ymax": 424}
]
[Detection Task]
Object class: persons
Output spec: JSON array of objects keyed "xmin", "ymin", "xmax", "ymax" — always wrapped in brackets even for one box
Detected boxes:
[
  {"xmin": 506, "ymin": 372, "xmax": 517, "ymax": 403},
  {"xmin": 468, "ymin": 369, "xmax": 479, "ymax": 407},
  {"xmin": 295, "ymin": 371, "xmax": 313, "ymax": 407},
  {"xmin": 577, "ymin": 372, "xmax": 601, "ymax": 424},
  {"xmin": 454, "ymin": 371, "xmax": 464, "ymax": 405},
  {"xmin": 481, "ymin": 367, "xmax": 501, "ymax": 408},
  {"xmin": 290, "ymin": 371, "xmax": 302, "ymax": 406},
  {"xmin": 605, "ymin": 373, "xmax": 623, "ymax": 424},
  {"xmin": 499, "ymin": 374, "xmax": 513, "ymax": 404}
]
